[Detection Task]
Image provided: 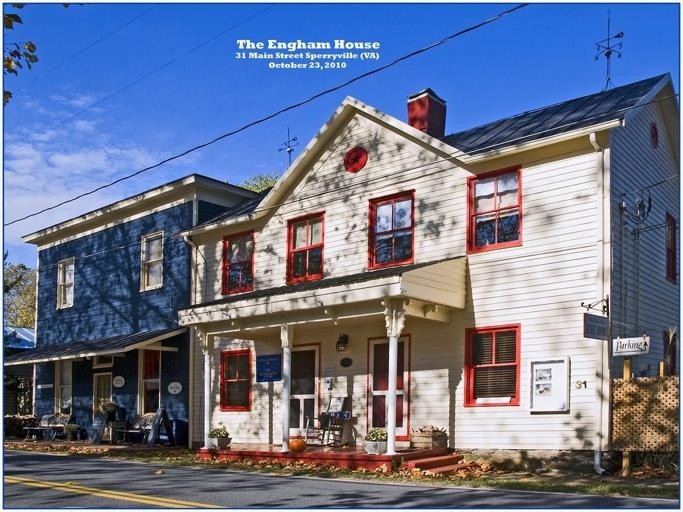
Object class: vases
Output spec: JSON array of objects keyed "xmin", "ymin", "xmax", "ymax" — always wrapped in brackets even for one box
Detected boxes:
[
  {"xmin": 363, "ymin": 441, "xmax": 386, "ymax": 455},
  {"xmin": 206, "ymin": 438, "xmax": 232, "ymax": 450}
]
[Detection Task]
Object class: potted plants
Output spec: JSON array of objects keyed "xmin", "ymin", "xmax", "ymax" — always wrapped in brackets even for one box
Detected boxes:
[{"xmin": 62, "ymin": 423, "xmax": 80, "ymax": 441}]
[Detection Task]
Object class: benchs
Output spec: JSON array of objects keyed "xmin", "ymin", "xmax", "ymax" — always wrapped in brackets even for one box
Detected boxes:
[
  {"xmin": 21, "ymin": 414, "xmax": 71, "ymax": 442},
  {"xmin": 113, "ymin": 412, "xmax": 164, "ymax": 447}
]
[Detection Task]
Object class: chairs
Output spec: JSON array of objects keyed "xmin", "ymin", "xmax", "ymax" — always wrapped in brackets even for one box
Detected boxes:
[{"xmin": 304, "ymin": 394, "xmax": 350, "ymax": 449}]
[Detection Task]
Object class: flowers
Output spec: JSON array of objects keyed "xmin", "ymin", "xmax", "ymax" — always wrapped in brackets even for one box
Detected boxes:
[
  {"xmin": 207, "ymin": 425, "xmax": 228, "ymax": 438},
  {"xmin": 364, "ymin": 428, "xmax": 387, "ymax": 441}
]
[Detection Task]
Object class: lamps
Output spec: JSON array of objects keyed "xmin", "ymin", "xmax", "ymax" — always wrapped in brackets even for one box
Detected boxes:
[{"xmin": 335, "ymin": 330, "xmax": 348, "ymax": 352}]
